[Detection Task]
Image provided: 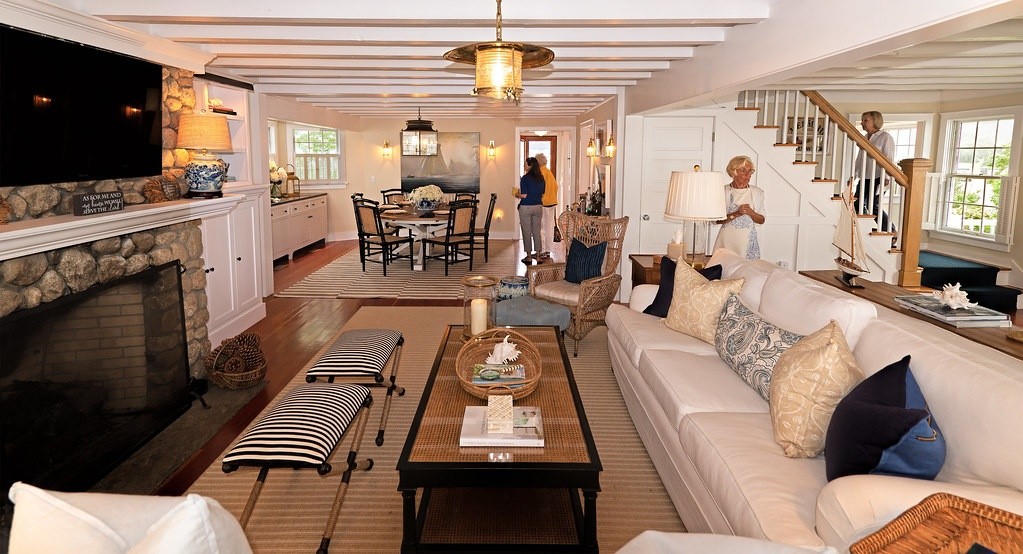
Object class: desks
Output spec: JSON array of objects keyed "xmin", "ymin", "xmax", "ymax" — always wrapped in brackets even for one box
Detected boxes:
[
  {"xmin": 376, "ymin": 202, "xmax": 470, "ymax": 270},
  {"xmin": 798, "ymin": 270, "xmax": 1023, "ymax": 361}
]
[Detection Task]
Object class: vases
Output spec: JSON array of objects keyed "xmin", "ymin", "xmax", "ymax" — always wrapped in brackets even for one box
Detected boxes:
[
  {"xmin": 271, "ymin": 184, "xmax": 281, "ymax": 197},
  {"xmin": 417, "ymin": 197, "xmax": 441, "ymax": 218}
]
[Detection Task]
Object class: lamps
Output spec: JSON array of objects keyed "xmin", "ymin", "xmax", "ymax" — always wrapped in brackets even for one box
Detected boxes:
[
  {"xmin": 442, "ymin": 0, "xmax": 555, "ymax": 106},
  {"xmin": 382, "ymin": 108, "xmax": 496, "ymax": 161},
  {"xmin": 586, "ymin": 138, "xmax": 613, "ymax": 158},
  {"xmin": 176, "ymin": 115, "xmax": 234, "ymax": 200},
  {"xmin": 665, "ymin": 165, "xmax": 727, "ymax": 269}
]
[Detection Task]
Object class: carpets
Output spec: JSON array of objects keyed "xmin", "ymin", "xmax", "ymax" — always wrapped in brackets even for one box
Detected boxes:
[
  {"xmin": 273, "ymin": 240, "xmax": 515, "ymax": 300},
  {"xmin": 182, "ymin": 305, "xmax": 686, "ymax": 554}
]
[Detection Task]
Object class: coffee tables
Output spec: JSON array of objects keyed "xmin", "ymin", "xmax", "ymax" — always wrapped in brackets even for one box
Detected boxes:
[{"xmin": 396, "ymin": 325, "xmax": 603, "ymax": 554}]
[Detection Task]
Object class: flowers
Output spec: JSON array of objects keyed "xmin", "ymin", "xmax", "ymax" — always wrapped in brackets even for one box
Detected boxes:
[{"xmin": 404, "ymin": 184, "xmax": 448, "ymax": 203}]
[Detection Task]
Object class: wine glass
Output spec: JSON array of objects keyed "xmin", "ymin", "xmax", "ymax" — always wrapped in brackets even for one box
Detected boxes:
[
  {"xmin": 411, "ymin": 199, "xmax": 417, "ymax": 214},
  {"xmin": 393, "ymin": 195, "xmax": 398, "ymax": 209},
  {"xmin": 404, "ymin": 192, "xmax": 409, "ymax": 207}
]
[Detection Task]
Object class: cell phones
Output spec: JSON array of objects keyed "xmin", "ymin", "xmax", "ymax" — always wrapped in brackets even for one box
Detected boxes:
[{"xmin": 0, "ymin": 23, "xmax": 163, "ymax": 186}]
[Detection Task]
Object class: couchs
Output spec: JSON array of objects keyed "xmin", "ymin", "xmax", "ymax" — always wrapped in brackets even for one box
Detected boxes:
[{"xmin": 604, "ymin": 248, "xmax": 1023, "ymax": 554}]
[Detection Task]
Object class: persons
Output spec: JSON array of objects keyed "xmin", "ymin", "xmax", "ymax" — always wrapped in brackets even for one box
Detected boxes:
[
  {"xmin": 516, "ymin": 158, "xmax": 545, "ymax": 263},
  {"xmin": 531, "ymin": 153, "xmax": 559, "ymax": 260},
  {"xmin": 711, "ymin": 155, "xmax": 766, "ymax": 262},
  {"xmin": 846, "ymin": 112, "xmax": 897, "ymax": 245}
]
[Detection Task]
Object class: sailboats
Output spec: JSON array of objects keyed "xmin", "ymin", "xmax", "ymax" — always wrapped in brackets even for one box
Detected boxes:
[{"xmin": 830, "ymin": 177, "xmax": 871, "ymax": 277}]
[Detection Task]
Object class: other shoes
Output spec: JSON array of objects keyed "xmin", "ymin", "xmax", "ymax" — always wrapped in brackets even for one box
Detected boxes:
[
  {"xmin": 546, "ymin": 252, "xmax": 550, "ymax": 257},
  {"xmin": 531, "ymin": 253, "xmax": 547, "ymax": 258}
]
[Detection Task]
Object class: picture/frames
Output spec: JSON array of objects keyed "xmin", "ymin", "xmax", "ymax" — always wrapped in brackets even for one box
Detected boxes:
[
  {"xmin": 594, "ymin": 165, "xmax": 610, "ymax": 208},
  {"xmin": 595, "ymin": 120, "xmax": 612, "ymax": 158}
]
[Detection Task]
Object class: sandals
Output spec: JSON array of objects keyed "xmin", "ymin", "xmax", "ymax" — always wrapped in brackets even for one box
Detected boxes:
[{"xmin": 892, "ymin": 229, "xmax": 898, "ymax": 245}]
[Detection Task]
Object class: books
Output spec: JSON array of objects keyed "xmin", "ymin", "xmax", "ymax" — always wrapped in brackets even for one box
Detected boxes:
[
  {"xmin": 460, "ymin": 405, "xmax": 545, "ymax": 447},
  {"xmin": 472, "ymin": 363, "xmax": 525, "ymax": 388}
]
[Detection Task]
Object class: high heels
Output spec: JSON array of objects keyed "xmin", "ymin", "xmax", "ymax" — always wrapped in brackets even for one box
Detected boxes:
[
  {"xmin": 521, "ymin": 257, "xmax": 532, "ymax": 266},
  {"xmin": 537, "ymin": 260, "xmax": 544, "ymax": 264}
]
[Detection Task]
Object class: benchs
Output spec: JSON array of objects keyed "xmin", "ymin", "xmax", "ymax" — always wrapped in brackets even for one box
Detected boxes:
[{"xmin": 222, "ymin": 329, "xmax": 406, "ymax": 554}]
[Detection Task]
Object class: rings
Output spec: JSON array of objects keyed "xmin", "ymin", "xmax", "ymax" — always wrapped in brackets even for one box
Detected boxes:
[{"xmin": 744, "ymin": 210, "xmax": 745, "ymax": 211}]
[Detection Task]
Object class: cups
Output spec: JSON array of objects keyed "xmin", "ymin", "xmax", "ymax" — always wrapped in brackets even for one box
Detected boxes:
[
  {"xmin": 462, "ymin": 276, "xmax": 498, "ymax": 339},
  {"xmin": 442, "ymin": 194, "xmax": 454, "ymax": 207}
]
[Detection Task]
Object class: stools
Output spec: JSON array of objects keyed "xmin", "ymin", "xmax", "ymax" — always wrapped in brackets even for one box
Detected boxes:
[{"xmin": 495, "ymin": 295, "xmax": 571, "ymax": 341}]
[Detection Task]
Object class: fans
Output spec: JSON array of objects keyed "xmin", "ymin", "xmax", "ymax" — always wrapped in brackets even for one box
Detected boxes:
[{"xmin": 269, "ymin": 160, "xmax": 288, "ymax": 185}]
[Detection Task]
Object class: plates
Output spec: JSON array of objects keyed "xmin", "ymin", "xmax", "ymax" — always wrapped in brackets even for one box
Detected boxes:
[
  {"xmin": 400, "ymin": 201, "xmax": 413, "ymax": 204},
  {"xmin": 384, "ymin": 210, "xmax": 405, "ymax": 213},
  {"xmin": 433, "ymin": 211, "xmax": 450, "ymax": 213},
  {"xmin": 379, "ymin": 205, "xmax": 398, "ymax": 208}
]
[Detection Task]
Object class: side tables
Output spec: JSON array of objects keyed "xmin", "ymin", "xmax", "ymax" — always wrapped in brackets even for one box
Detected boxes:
[{"xmin": 628, "ymin": 254, "xmax": 712, "ymax": 289}]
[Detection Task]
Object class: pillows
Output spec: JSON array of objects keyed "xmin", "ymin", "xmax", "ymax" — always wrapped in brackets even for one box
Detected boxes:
[
  {"xmin": 564, "ymin": 237, "xmax": 608, "ymax": 283},
  {"xmin": 642, "ymin": 259, "xmax": 948, "ymax": 482}
]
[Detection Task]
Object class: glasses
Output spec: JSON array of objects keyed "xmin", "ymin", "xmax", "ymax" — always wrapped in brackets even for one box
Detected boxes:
[{"xmin": 735, "ymin": 169, "xmax": 755, "ymax": 175}]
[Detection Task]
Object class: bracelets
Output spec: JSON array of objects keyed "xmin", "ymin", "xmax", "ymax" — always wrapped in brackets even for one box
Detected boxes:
[{"xmin": 730, "ymin": 213, "xmax": 735, "ymax": 219}]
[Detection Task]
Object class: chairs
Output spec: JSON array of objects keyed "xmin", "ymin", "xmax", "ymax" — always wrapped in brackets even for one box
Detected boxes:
[
  {"xmin": 351, "ymin": 189, "xmax": 497, "ymax": 276},
  {"xmin": 527, "ymin": 210, "xmax": 629, "ymax": 355}
]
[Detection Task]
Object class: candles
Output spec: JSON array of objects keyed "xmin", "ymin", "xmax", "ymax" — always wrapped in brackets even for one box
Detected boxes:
[{"xmin": 471, "ymin": 299, "xmax": 488, "ymax": 336}]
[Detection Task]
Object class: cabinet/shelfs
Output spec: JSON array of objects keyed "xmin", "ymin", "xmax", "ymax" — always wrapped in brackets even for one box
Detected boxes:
[
  {"xmin": 193, "ymin": 78, "xmax": 266, "ymax": 353},
  {"xmin": 270, "ymin": 195, "xmax": 327, "ymax": 260}
]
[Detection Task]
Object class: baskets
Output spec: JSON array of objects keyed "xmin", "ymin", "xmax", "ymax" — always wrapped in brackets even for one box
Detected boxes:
[
  {"xmin": 207, "ymin": 340, "xmax": 268, "ymax": 390},
  {"xmin": 456, "ymin": 328, "xmax": 542, "ymax": 399},
  {"xmin": 849, "ymin": 492, "xmax": 1023, "ymax": 554}
]
